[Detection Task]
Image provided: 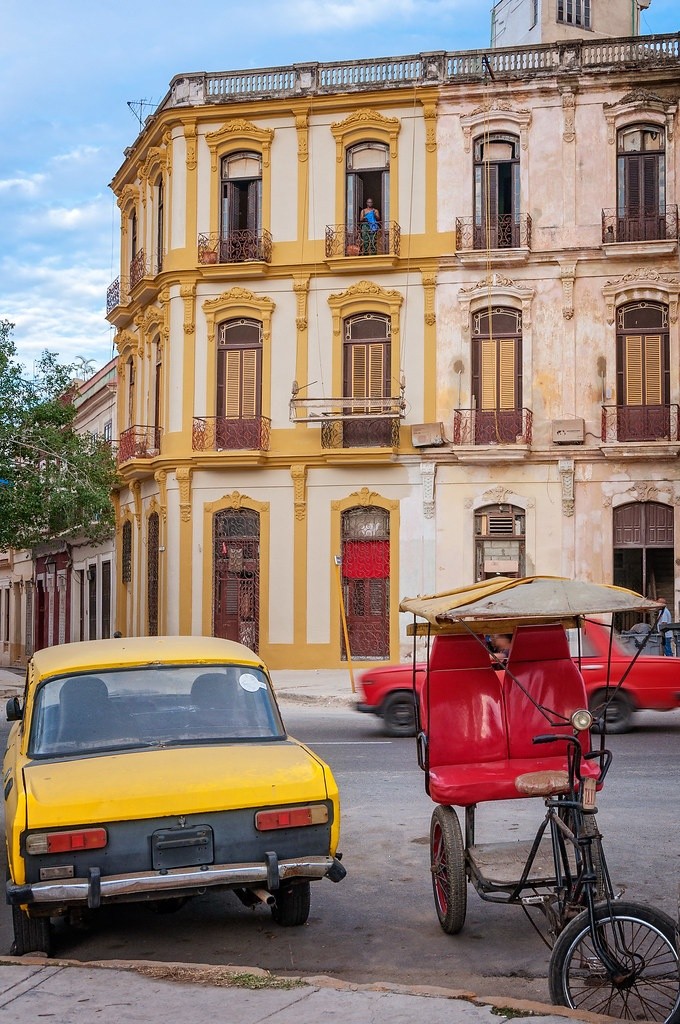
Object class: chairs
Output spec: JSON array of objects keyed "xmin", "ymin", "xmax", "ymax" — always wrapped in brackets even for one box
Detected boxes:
[
  {"xmin": 190, "ymin": 673, "xmax": 243, "ymax": 737},
  {"xmin": 59, "ymin": 678, "xmax": 111, "ymax": 742}
]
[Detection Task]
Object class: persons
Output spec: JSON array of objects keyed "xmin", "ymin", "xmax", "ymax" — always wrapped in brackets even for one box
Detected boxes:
[
  {"xmin": 487, "ymin": 633, "xmax": 515, "ymax": 668},
  {"xmin": 657, "ymin": 598, "xmax": 673, "ymax": 656}
]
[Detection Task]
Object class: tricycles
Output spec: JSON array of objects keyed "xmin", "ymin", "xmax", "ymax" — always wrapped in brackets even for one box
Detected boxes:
[{"xmin": 400, "ymin": 574, "xmax": 680, "ymax": 1024}]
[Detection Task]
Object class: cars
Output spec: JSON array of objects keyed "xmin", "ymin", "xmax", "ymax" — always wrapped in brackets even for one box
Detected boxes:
[
  {"xmin": 0, "ymin": 636, "xmax": 348, "ymax": 962},
  {"xmin": 354, "ymin": 618, "xmax": 680, "ymax": 735}
]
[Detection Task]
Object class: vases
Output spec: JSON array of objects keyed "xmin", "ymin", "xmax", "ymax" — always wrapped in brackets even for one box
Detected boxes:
[
  {"xmin": 347, "ymin": 243, "xmax": 361, "ymax": 256},
  {"xmin": 204, "ymin": 251, "xmax": 217, "ymax": 264}
]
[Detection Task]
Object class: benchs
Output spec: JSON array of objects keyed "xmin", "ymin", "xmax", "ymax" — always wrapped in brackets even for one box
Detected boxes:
[{"xmin": 414, "ymin": 625, "xmax": 604, "ymax": 805}]
